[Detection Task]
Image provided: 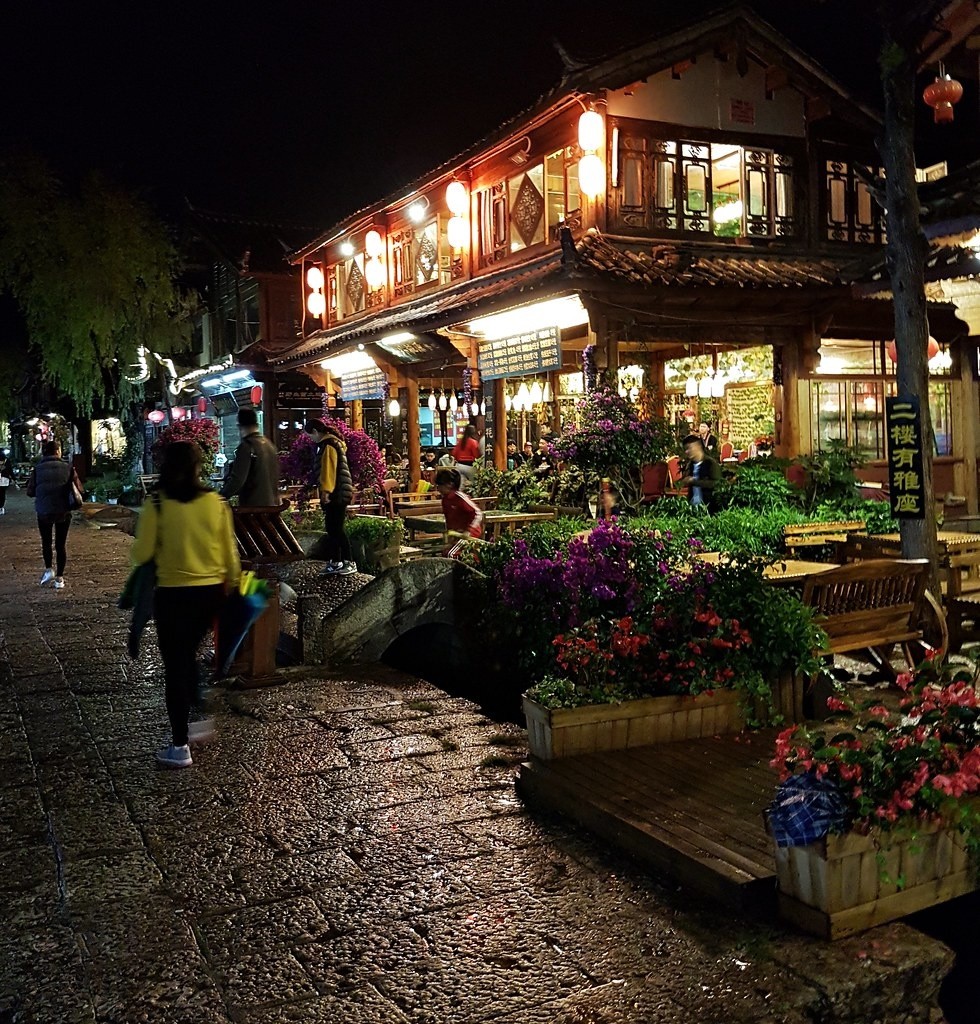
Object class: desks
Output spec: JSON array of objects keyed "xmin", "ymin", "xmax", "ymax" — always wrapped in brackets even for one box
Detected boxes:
[
  {"xmin": 407, "ymin": 508, "xmax": 535, "ymax": 538},
  {"xmin": 844, "ymin": 531, "xmax": 980, "ymax": 584},
  {"xmin": 636, "ymin": 548, "xmax": 843, "ymax": 593}
]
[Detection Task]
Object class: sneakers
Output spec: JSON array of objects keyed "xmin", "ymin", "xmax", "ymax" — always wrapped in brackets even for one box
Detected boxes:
[
  {"xmin": 54, "ymin": 579, "xmax": 65, "ymax": 589},
  {"xmin": 185, "ymin": 714, "xmax": 216, "ymax": 740},
  {"xmin": 338, "ymin": 561, "xmax": 358, "ymax": 575},
  {"xmin": 157, "ymin": 743, "xmax": 193, "ymax": 767},
  {"xmin": 319, "ymin": 560, "xmax": 345, "ymax": 575},
  {"xmin": 40, "ymin": 569, "xmax": 55, "ymax": 585}
]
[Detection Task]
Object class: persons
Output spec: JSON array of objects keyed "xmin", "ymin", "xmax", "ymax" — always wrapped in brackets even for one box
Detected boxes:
[
  {"xmin": 424, "ymin": 448, "xmax": 438, "ymax": 469},
  {"xmin": 698, "ymin": 421, "xmax": 720, "ymax": 461},
  {"xmin": 452, "ymin": 425, "xmax": 482, "ymax": 490},
  {"xmin": 306, "ymin": 419, "xmax": 359, "ymax": 575},
  {"xmin": 0, "ymin": 447, "xmax": 22, "ymax": 515},
  {"xmin": 26, "ymin": 441, "xmax": 86, "ymax": 588},
  {"xmin": 438, "ymin": 449, "xmax": 457, "ymax": 466},
  {"xmin": 221, "ymin": 406, "xmax": 282, "ymax": 508},
  {"xmin": 506, "ymin": 423, "xmax": 560, "ymax": 482},
  {"xmin": 379, "ymin": 445, "xmax": 402, "ymax": 467},
  {"xmin": 683, "ymin": 435, "xmax": 726, "ymax": 516},
  {"xmin": 436, "ymin": 467, "xmax": 484, "ymax": 565},
  {"xmin": 131, "ymin": 444, "xmax": 242, "ymax": 765}
]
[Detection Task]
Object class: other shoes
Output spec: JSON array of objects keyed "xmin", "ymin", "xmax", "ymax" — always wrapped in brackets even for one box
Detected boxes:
[{"xmin": 0, "ymin": 507, "xmax": 5, "ymax": 514}]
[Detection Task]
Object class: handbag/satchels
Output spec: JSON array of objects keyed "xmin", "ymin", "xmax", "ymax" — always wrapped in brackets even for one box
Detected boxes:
[
  {"xmin": 66, "ymin": 468, "xmax": 83, "ymax": 512},
  {"xmin": 0, "ymin": 477, "xmax": 9, "ymax": 486},
  {"xmin": 116, "ymin": 492, "xmax": 160, "ymax": 610}
]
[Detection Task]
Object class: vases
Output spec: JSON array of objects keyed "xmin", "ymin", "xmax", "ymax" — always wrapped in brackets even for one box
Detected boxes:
[
  {"xmin": 769, "ymin": 792, "xmax": 979, "ymax": 940},
  {"xmin": 518, "ymin": 681, "xmax": 752, "ymax": 759}
]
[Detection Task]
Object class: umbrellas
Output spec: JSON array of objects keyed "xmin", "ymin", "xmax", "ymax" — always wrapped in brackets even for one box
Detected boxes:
[{"xmin": 215, "ymin": 568, "xmax": 271, "ymax": 679}]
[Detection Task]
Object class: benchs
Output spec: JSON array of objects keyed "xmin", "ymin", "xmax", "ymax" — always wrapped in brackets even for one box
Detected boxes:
[
  {"xmin": 944, "ymin": 537, "xmax": 980, "ymax": 657},
  {"xmin": 779, "ymin": 520, "xmax": 872, "ymax": 561},
  {"xmin": 800, "ymin": 555, "xmax": 949, "ymax": 679},
  {"xmin": 134, "ymin": 475, "xmax": 558, "ymax": 570}
]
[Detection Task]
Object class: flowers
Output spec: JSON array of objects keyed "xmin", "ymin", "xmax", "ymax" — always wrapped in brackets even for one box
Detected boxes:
[
  {"xmin": 768, "ymin": 652, "xmax": 979, "ymax": 868},
  {"xmin": 547, "ymin": 587, "xmax": 788, "ymax": 731}
]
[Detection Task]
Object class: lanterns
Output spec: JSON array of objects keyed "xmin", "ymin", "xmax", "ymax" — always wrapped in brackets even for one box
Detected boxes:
[
  {"xmin": 921, "ymin": 74, "xmax": 964, "ymax": 124},
  {"xmin": 444, "ymin": 182, "xmax": 468, "ymax": 252},
  {"xmin": 365, "ymin": 229, "xmax": 386, "ymax": 286},
  {"xmin": 576, "ymin": 108, "xmax": 605, "ymax": 202},
  {"xmin": 888, "ymin": 335, "xmax": 939, "ymax": 362},
  {"xmin": 251, "ymin": 385, "xmax": 262, "ymax": 406},
  {"xmin": 198, "ymin": 396, "xmax": 207, "ymax": 416},
  {"xmin": 170, "ymin": 406, "xmax": 185, "ymax": 420},
  {"xmin": 306, "ymin": 266, "xmax": 326, "ymax": 319},
  {"xmin": 148, "ymin": 410, "xmax": 165, "ymax": 423}
]
